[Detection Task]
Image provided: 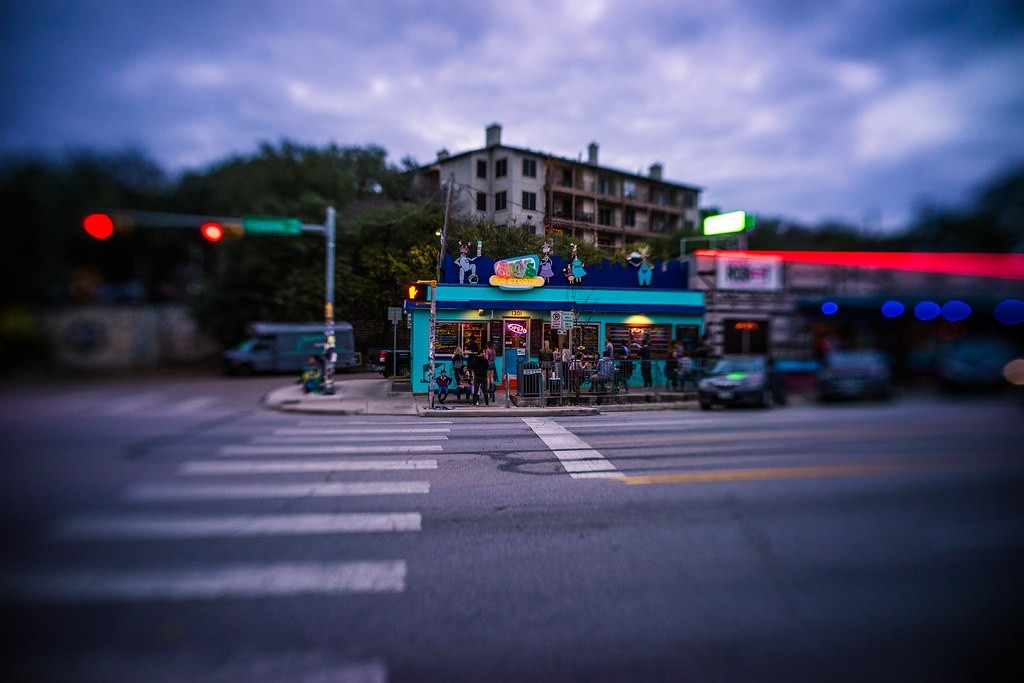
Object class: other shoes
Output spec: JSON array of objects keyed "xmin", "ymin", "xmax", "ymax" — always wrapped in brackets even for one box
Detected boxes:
[
  {"xmin": 472, "ymin": 401, "xmax": 477, "ymax": 406},
  {"xmin": 666, "ymin": 386, "xmax": 670, "ymax": 391},
  {"xmin": 485, "ymin": 402, "xmax": 489, "ymax": 405},
  {"xmin": 492, "ymin": 399, "xmax": 495, "ymax": 402},
  {"xmin": 573, "ymin": 388, "xmax": 577, "ymax": 392},
  {"xmin": 673, "ymin": 388, "xmax": 678, "ymax": 391},
  {"xmin": 589, "ymin": 389, "xmax": 593, "ymax": 392},
  {"xmin": 642, "ymin": 387, "xmax": 649, "ymax": 390},
  {"xmin": 466, "ymin": 399, "xmax": 469, "ymax": 403},
  {"xmin": 650, "ymin": 387, "xmax": 656, "ymax": 391},
  {"xmin": 562, "ymin": 386, "xmax": 567, "ymax": 390}
]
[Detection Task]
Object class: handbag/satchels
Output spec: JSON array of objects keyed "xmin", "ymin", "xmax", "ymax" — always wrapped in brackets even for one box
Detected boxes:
[{"xmin": 459, "ymin": 381, "xmax": 471, "ymax": 387}]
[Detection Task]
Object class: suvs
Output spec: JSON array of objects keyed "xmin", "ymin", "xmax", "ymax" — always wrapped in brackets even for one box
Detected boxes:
[{"xmin": 698, "ymin": 353, "xmax": 788, "ymax": 410}]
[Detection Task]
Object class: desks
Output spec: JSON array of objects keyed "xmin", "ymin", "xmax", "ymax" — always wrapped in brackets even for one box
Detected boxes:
[{"xmin": 581, "ymin": 369, "xmax": 597, "ymax": 390}]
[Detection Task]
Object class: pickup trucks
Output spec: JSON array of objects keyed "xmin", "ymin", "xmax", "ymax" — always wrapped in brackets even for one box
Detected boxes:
[{"xmin": 368, "ymin": 346, "xmax": 412, "ymax": 377}]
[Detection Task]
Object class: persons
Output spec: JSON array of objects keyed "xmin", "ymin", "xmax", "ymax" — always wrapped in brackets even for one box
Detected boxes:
[
  {"xmin": 436, "ymin": 340, "xmax": 496, "ymax": 405},
  {"xmin": 538, "ymin": 336, "xmax": 698, "ymax": 394}
]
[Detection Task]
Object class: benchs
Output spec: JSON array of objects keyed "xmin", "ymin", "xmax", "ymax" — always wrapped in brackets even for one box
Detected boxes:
[{"xmin": 570, "ymin": 378, "xmax": 629, "ymax": 392}]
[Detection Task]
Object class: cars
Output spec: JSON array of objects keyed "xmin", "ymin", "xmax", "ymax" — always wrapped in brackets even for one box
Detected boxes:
[{"xmin": 814, "ymin": 360, "xmax": 883, "ymax": 400}]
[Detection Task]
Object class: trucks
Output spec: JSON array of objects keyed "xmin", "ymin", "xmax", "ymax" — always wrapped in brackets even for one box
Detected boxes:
[{"xmin": 223, "ymin": 323, "xmax": 356, "ymax": 373}]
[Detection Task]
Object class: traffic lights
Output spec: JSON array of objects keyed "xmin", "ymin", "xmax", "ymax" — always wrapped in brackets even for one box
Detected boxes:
[
  {"xmin": 83, "ymin": 215, "xmax": 115, "ymax": 239},
  {"xmin": 202, "ymin": 221, "xmax": 231, "ymax": 242}
]
